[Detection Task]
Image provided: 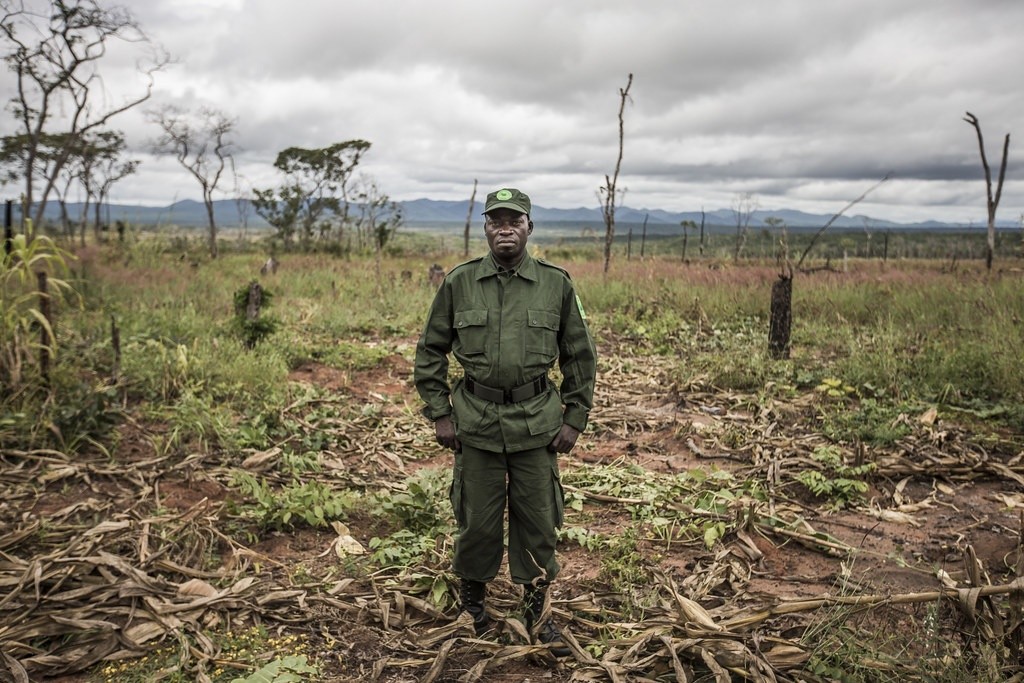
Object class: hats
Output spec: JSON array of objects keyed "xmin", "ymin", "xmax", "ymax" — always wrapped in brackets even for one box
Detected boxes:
[{"xmin": 481, "ymin": 188, "xmax": 532, "ymax": 215}]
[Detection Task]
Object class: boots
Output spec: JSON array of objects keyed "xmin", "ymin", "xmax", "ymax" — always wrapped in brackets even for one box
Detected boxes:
[
  {"xmin": 435, "ymin": 576, "xmax": 487, "ymax": 641},
  {"xmin": 522, "ymin": 581, "xmax": 574, "ymax": 657}
]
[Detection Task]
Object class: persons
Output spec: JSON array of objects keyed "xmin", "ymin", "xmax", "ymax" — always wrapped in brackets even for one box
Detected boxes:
[{"xmin": 410, "ymin": 187, "xmax": 598, "ymax": 629}]
